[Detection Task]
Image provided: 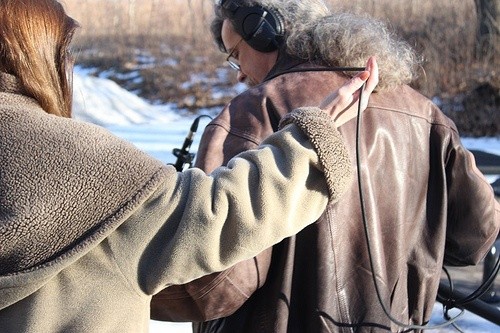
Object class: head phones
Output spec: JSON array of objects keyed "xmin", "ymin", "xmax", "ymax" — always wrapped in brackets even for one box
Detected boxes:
[{"xmin": 221, "ymin": 0, "xmax": 286, "ymax": 52}]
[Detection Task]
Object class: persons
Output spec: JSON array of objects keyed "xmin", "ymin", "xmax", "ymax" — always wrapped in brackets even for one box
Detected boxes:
[
  {"xmin": 151, "ymin": 0, "xmax": 500, "ymax": 333},
  {"xmin": 0, "ymin": 0, "xmax": 379, "ymax": 333}
]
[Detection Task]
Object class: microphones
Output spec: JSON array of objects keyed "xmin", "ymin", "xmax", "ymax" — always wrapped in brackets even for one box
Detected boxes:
[{"xmin": 168, "ymin": 117, "xmax": 199, "ymax": 172}]
